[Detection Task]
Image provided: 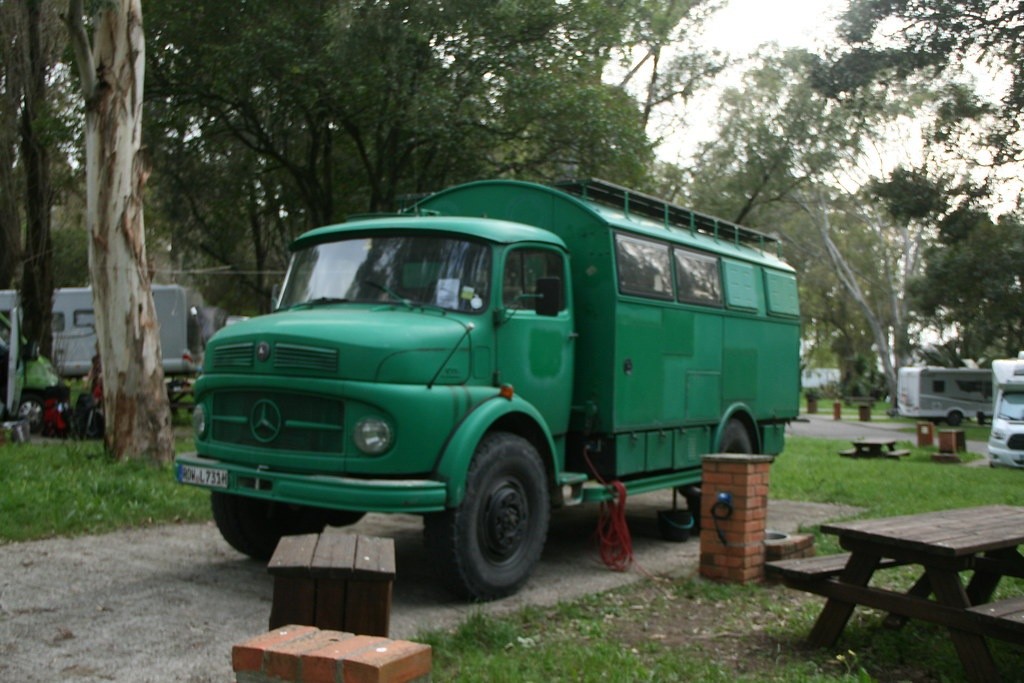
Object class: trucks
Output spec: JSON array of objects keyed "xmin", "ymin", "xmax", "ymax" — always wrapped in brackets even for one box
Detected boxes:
[
  {"xmin": 0, "ymin": 285, "xmax": 250, "ymax": 437},
  {"xmin": 989, "ymin": 359, "xmax": 1024, "ymax": 469},
  {"xmin": 175, "ymin": 175, "xmax": 801, "ymax": 608},
  {"xmin": 897, "ymin": 365, "xmax": 994, "ymax": 427}
]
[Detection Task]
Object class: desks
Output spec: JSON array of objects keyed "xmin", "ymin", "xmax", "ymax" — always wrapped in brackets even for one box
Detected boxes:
[
  {"xmin": 166, "ymin": 371, "xmax": 197, "ymax": 405},
  {"xmin": 805, "ymin": 505, "xmax": 1024, "ymax": 683},
  {"xmin": 850, "ymin": 440, "xmax": 900, "ymax": 461},
  {"xmin": 845, "ymin": 396, "xmax": 876, "ymax": 408}
]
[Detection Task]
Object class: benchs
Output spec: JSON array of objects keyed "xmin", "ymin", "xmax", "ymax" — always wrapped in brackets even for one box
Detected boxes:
[
  {"xmin": 765, "ymin": 553, "xmax": 908, "ymax": 592},
  {"xmin": 169, "ymin": 402, "xmax": 194, "ymax": 409},
  {"xmin": 886, "ymin": 449, "xmax": 911, "ymax": 458},
  {"xmin": 957, "ymin": 596, "xmax": 1024, "ymax": 644},
  {"xmin": 839, "ymin": 448, "xmax": 868, "ymax": 456}
]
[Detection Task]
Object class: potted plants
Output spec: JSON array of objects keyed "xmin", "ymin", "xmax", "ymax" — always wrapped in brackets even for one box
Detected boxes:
[{"xmin": 805, "ymin": 388, "xmax": 822, "ymax": 412}]
